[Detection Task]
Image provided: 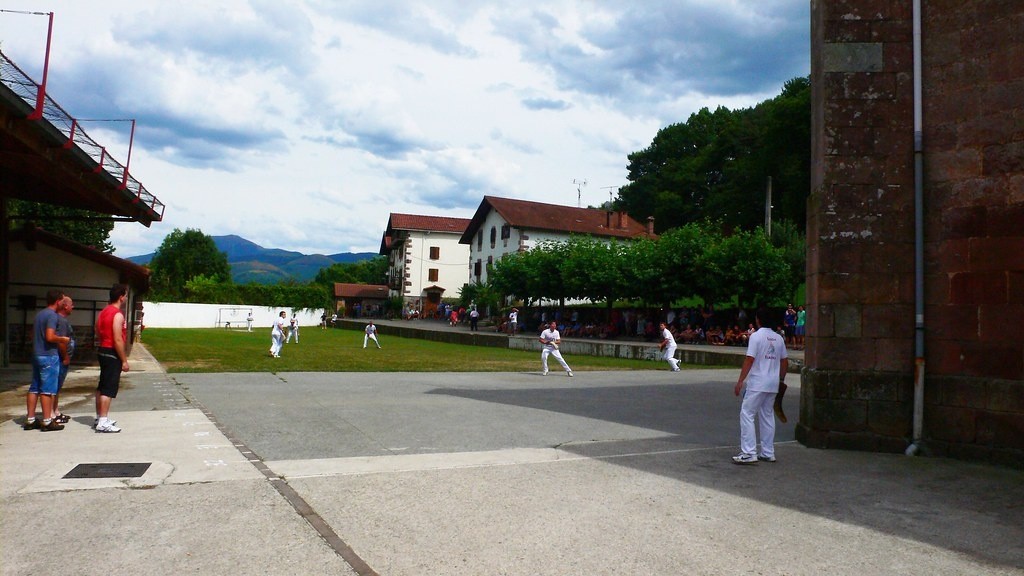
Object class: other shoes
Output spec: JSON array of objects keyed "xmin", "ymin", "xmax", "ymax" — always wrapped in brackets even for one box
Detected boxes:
[
  {"xmin": 672, "ymin": 367, "xmax": 680, "ymax": 372},
  {"xmin": 53, "ymin": 415, "xmax": 69, "ymax": 423},
  {"xmin": 676, "ymin": 360, "xmax": 681, "ymax": 367},
  {"xmin": 567, "ymin": 371, "xmax": 573, "ymax": 377},
  {"xmin": 57, "ymin": 413, "xmax": 70, "ymax": 419},
  {"xmin": 274, "ymin": 356, "xmax": 280, "ymax": 358},
  {"xmin": 378, "ymin": 346, "xmax": 381, "ymax": 349},
  {"xmin": 543, "ymin": 370, "xmax": 549, "ymax": 376}
]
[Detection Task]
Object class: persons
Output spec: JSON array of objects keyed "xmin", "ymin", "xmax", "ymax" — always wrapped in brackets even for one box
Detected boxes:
[
  {"xmin": 351, "ymin": 295, "xmax": 806, "ymax": 351},
  {"xmin": 732, "ymin": 309, "xmax": 788, "ymax": 463},
  {"xmin": 93, "ymin": 284, "xmax": 129, "ymax": 433},
  {"xmin": 23, "ymin": 290, "xmax": 70, "ymax": 432},
  {"xmin": 657, "ymin": 322, "xmax": 681, "ymax": 372},
  {"xmin": 48, "ymin": 295, "xmax": 75, "ymax": 423},
  {"xmin": 330, "ymin": 312, "xmax": 337, "ymax": 328},
  {"xmin": 246, "ymin": 313, "xmax": 255, "ymax": 332},
  {"xmin": 539, "ymin": 321, "xmax": 573, "ymax": 377},
  {"xmin": 290, "ymin": 313, "xmax": 299, "ymax": 328},
  {"xmin": 285, "ymin": 320, "xmax": 299, "ymax": 343},
  {"xmin": 320, "ymin": 313, "xmax": 327, "ymax": 329},
  {"xmin": 269, "ymin": 311, "xmax": 286, "ymax": 358}
]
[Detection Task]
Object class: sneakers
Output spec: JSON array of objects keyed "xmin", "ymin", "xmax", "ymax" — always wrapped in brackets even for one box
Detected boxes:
[
  {"xmin": 41, "ymin": 419, "xmax": 64, "ymax": 432},
  {"xmin": 95, "ymin": 422, "xmax": 122, "ymax": 433},
  {"xmin": 757, "ymin": 454, "xmax": 776, "ymax": 462},
  {"xmin": 732, "ymin": 454, "xmax": 758, "ymax": 464},
  {"xmin": 94, "ymin": 418, "xmax": 116, "ymax": 427},
  {"xmin": 23, "ymin": 418, "xmax": 41, "ymax": 430}
]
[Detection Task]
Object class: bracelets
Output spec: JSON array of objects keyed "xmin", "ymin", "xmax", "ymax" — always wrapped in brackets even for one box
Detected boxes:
[
  {"xmin": 780, "ymin": 378, "xmax": 784, "ymax": 380},
  {"xmin": 738, "ymin": 380, "xmax": 743, "ymax": 383}
]
